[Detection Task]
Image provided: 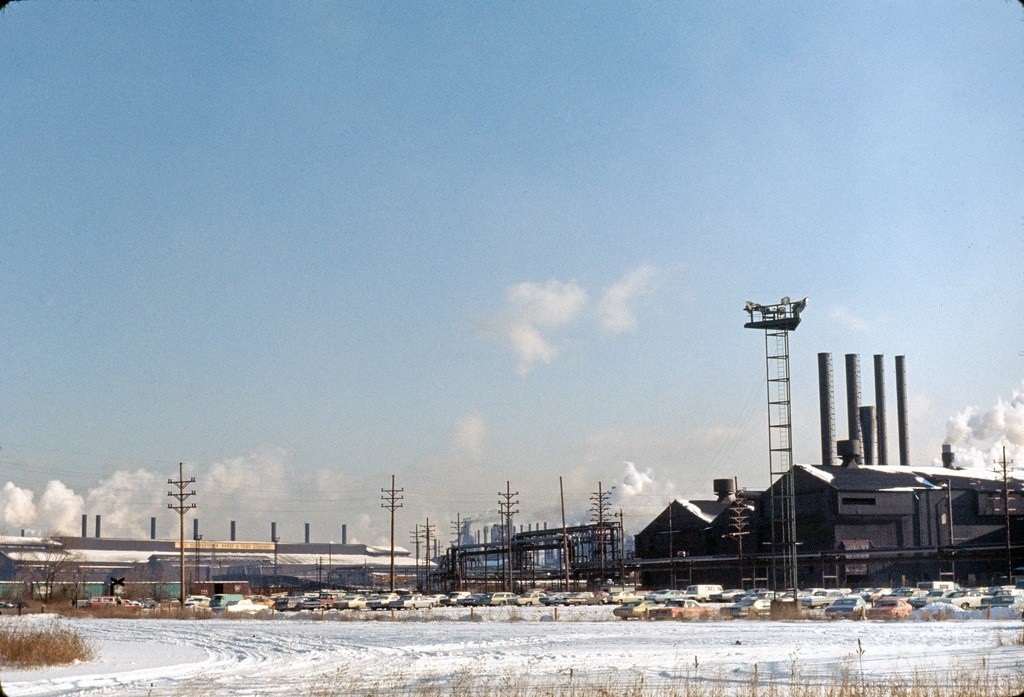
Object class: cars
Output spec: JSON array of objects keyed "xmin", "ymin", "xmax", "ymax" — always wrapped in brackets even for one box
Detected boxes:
[
  {"xmin": 865, "ymin": 597, "xmax": 914, "ymax": 622},
  {"xmin": 69, "ymin": 579, "xmax": 1024, "ymax": 620},
  {"xmin": 824, "ymin": 594, "xmax": 867, "ymax": 621},
  {"xmin": 0, "ymin": 599, "xmax": 30, "ymax": 609}
]
